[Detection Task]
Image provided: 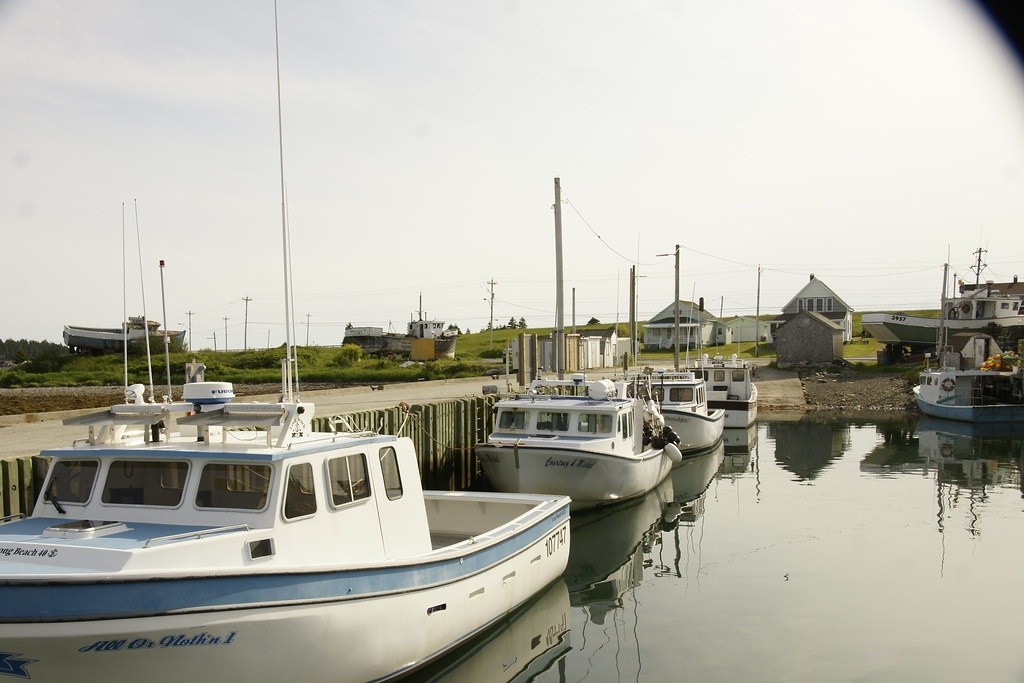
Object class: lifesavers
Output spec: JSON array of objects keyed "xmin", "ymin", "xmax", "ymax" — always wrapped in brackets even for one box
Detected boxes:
[
  {"xmin": 962, "ymin": 304, "xmax": 970, "ymax": 313},
  {"xmin": 941, "ymin": 378, "xmax": 956, "ymax": 392}
]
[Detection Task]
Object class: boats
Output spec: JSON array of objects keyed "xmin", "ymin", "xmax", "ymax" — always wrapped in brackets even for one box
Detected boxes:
[
  {"xmin": 396, "ymin": 580, "xmax": 575, "ymax": 683},
  {"xmin": 0, "ymin": 1, "xmax": 577, "ymax": 683},
  {"xmin": 912, "ymin": 414, "xmax": 1024, "ymax": 534},
  {"xmin": 912, "ymin": 243, "xmax": 1024, "ymax": 425},
  {"xmin": 681, "ymin": 350, "xmax": 760, "ymax": 428},
  {"xmin": 721, "ymin": 421, "xmax": 760, "ymax": 495},
  {"xmin": 653, "ymin": 437, "xmax": 730, "ymax": 579},
  {"xmin": 641, "ymin": 370, "xmax": 727, "ymax": 455},
  {"xmin": 560, "ymin": 478, "xmax": 680, "ymax": 626},
  {"xmin": 466, "ymin": 367, "xmax": 686, "ymax": 516},
  {"xmin": 859, "ymin": 246, "xmax": 1024, "ymax": 348}
]
